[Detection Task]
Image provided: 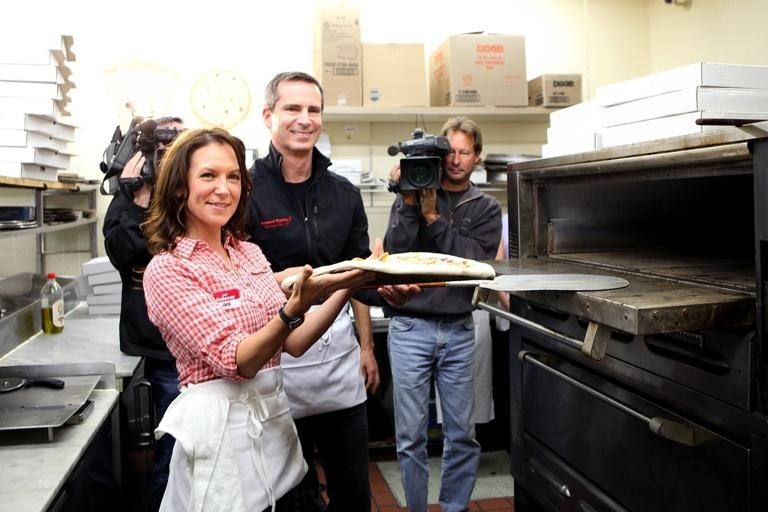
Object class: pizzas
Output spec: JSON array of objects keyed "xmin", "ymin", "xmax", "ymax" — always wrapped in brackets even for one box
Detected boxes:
[{"xmin": 281, "ymin": 253, "xmax": 495, "ymax": 294}]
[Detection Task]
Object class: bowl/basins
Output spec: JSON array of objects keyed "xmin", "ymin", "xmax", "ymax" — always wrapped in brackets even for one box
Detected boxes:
[{"xmin": 0, "ymin": 206, "xmax": 37, "ymax": 222}]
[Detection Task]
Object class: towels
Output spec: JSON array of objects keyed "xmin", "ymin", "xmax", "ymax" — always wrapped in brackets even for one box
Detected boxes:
[{"xmin": 435, "ymin": 309, "xmax": 495, "ymax": 425}]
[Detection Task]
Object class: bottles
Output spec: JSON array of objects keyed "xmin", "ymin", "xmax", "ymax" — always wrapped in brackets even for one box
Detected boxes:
[{"xmin": 40, "ymin": 272, "xmax": 65, "ymax": 336}]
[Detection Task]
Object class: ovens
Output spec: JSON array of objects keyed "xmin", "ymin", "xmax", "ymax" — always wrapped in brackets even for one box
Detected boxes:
[{"xmin": 471, "ymin": 121, "xmax": 768, "ymax": 512}]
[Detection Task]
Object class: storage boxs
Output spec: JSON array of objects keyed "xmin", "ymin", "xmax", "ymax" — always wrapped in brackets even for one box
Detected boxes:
[
  {"xmin": 526, "ymin": 73, "xmax": 582, "ymax": 108},
  {"xmin": 361, "ymin": 43, "xmax": 428, "ymax": 109},
  {"xmin": 310, "ymin": 3, "xmax": 363, "ymax": 106},
  {"xmin": 429, "ymin": 30, "xmax": 528, "ymax": 109}
]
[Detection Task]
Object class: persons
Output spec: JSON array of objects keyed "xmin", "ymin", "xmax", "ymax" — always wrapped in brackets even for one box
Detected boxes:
[
  {"xmin": 494, "ymin": 214, "xmax": 514, "ymax": 350},
  {"xmin": 104, "ymin": 115, "xmax": 184, "ymax": 502},
  {"xmin": 435, "ymin": 279, "xmax": 495, "ymax": 433},
  {"xmin": 383, "ymin": 117, "xmax": 501, "ymax": 512},
  {"xmin": 143, "ymin": 128, "xmax": 376, "ymax": 512},
  {"xmin": 233, "ymin": 69, "xmax": 427, "ymax": 512}
]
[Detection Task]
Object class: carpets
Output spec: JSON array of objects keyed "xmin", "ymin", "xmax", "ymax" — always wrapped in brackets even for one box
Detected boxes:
[{"xmin": 375, "ymin": 450, "xmax": 514, "ymax": 509}]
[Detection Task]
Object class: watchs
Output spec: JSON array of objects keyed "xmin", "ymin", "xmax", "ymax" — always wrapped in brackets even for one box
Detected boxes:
[{"xmin": 279, "ymin": 308, "xmax": 305, "ymax": 330}]
